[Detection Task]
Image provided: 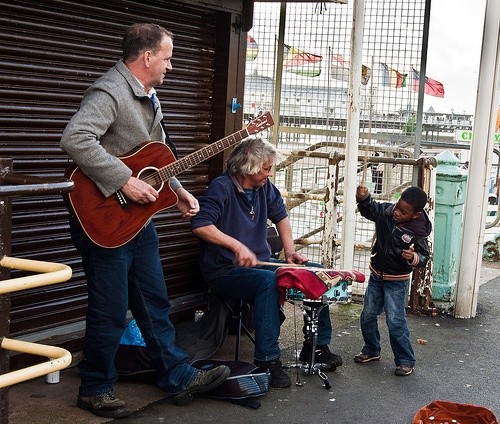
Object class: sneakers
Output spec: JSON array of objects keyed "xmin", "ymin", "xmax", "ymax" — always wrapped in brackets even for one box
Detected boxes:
[
  {"xmin": 395, "ymin": 364, "xmax": 412, "ymax": 375},
  {"xmin": 253, "ymin": 359, "xmax": 292, "ymax": 387},
  {"xmin": 171, "ymin": 365, "xmax": 230, "ymax": 405},
  {"xmin": 354, "ymin": 351, "xmax": 381, "ymax": 363},
  {"xmin": 299, "ymin": 344, "xmax": 342, "ymax": 366},
  {"xmin": 77, "ymin": 390, "xmax": 132, "ymax": 417}
]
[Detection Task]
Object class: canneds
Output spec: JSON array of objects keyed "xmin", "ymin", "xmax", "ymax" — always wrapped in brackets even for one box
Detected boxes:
[
  {"xmin": 195, "ymin": 310, "xmax": 204, "ymax": 322},
  {"xmin": 46, "ymin": 357, "xmax": 60, "ymax": 383}
]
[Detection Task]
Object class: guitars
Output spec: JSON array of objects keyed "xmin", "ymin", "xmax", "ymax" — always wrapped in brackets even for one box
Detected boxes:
[{"xmin": 64, "ymin": 111, "xmax": 275, "ymax": 249}]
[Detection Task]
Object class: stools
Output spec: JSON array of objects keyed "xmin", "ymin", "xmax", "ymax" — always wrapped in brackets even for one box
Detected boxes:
[{"xmin": 210, "ymin": 284, "xmax": 255, "ymax": 361}]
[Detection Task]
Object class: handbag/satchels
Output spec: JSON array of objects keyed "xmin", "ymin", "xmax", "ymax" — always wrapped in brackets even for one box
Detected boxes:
[{"xmin": 187, "ymin": 359, "xmax": 270, "ymax": 411}]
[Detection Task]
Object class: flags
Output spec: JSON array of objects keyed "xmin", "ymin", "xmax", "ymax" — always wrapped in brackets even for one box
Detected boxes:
[{"xmin": 282, "ymin": 44, "xmax": 445, "ymax": 98}]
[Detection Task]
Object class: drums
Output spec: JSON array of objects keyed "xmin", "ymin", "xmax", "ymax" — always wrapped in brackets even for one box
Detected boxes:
[{"xmin": 273, "ymin": 265, "xmax": 354, "ymax": 304}]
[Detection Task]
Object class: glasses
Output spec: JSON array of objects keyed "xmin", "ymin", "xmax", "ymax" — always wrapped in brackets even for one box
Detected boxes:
[{"xmin": 261, "ymin": 165, "xmax": 273, "ymax": 173}]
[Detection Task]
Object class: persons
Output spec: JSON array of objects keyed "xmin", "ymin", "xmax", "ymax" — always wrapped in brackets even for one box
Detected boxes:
[
  {"xmin": 192, "ymin": 137, "xmax": 341, "ymax": 388},
  {"xmin": 61, "ymin": 23, "xmax": 230, "ymax": 419},
  {"xmin": 353, "ymin": 184, "xmax": 432, "ymax": 376}
]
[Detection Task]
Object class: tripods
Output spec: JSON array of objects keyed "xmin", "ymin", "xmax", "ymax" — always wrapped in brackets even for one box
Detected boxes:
[{"xmin": 283, "ymin": 300, "xmax": 338, "ymax": 389}]
